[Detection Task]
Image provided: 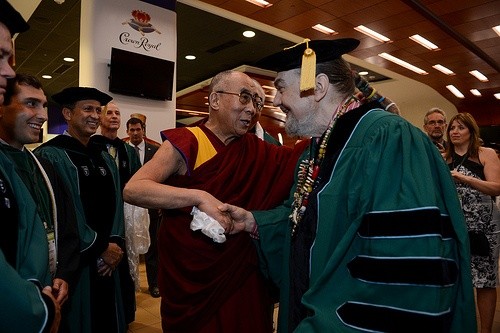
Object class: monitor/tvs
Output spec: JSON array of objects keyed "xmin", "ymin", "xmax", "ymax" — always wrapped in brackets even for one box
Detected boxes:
[{"xmin": 109, "ymin": 47, "xmax": 174, "ymax": 101}]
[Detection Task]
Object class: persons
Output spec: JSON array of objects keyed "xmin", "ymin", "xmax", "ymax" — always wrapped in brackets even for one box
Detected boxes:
[
  {"xmin": 0, "ymin": 0, "xmax": 137, "ymax": 333},
  {"xmin": 122, "ymin": 39, "xmax": 478, "ymax": 333},
  {"xmin": 423, "ymin": 107, "xmax": 500, "ymax": 333}
]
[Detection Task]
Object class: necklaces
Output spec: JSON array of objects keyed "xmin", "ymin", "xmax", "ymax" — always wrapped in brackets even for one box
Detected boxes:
[
  {"xmin": 452, "ymin": 152, "xmax": 468, "ymax": 172},
  {"xmin": 288, "ymin": 94, "xmax": 356, "ymax": 237}
]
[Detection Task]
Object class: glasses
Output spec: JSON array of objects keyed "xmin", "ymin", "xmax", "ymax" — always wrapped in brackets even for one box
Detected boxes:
[{"xmin": 216, "ymin": 91, "xmax": 261, "ymax": 109}]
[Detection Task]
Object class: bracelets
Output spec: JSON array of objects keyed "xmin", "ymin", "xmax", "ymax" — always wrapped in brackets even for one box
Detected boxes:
[{"xmin": 249, "ymin": 221, "xmax": 259, "ymax": 239}]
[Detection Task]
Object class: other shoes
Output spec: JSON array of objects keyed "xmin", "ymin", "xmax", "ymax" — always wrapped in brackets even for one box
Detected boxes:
[{"xmin": 150, "ymin": 287, "xmax": 160, "ymax": 297}]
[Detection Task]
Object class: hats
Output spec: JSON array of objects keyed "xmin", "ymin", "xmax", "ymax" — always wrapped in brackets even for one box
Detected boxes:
[
  {"xmin": 254, "ymin": 38, "xmax": 360, "ymax": 93},
  {"xmin": 52, "ymin": 86, "xmax": 113, "ymax": 117}
]
